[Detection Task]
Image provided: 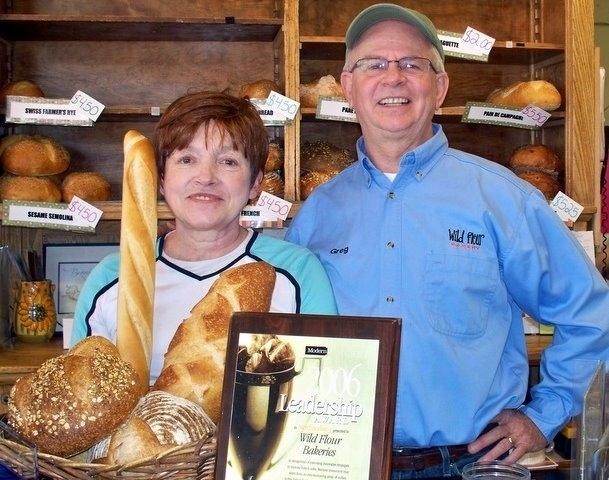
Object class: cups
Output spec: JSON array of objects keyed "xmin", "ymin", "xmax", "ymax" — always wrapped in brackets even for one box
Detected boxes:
[
  {"xmin": 460, "ymin": 458, "xmax": 532, "ymax": 480},
  {"xmin": 226, "ymin": 357, "xmax": 296, "ymax": 480}
]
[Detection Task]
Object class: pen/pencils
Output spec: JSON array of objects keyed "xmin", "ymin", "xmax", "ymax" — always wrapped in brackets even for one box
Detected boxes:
[{"xmin": 5, "ymin": 245, "xmax": 42, "ymax": 281}]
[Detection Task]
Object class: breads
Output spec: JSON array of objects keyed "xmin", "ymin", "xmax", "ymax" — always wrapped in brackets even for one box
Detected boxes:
[
  {"xmin": 92, "ymin": 417, "xmax": 176, "ymax": 463},
  {"xmin": 300, "ymin": 137, "xmax": 354, "ymax": 199},
  {"xmin": 153, "ymin": 261, "xmax": 276, "ymax": 424},
  {"xmin": 3, "ymin": 173, "xmax": 62, "ymax": 203},
  {"xmin": 62, "ymin": 172, "xmax": 111, "ymax": 203},
  {"xmin": 117, "ymin": 130, "xmax": 159, "ymax": 389},
  {"xmin": 249, "ymin": 137, "xmax": 286, "ymax": 205},
  {"xmin": 299, "ymin": 74, "xmax": 345, "ymax": 108},
  {"xmin": 486, "ymin": 80, "xmax": 562, "ymax": 110},
  {"xmin": 7, "ymin": 335, "xmax": 140, "ymax": 458},
  {"xmin": 511, "ymin": 144, "xmax": 557, "ymax": 199},
  {"xmin": 2, "ymin": 135, "xmax": 71, "ymax": 175},
  {"xmin": 240, "ymin": 78, "xmax": 282, "ymax": 99},
  {"xmin": 1, "ymin": 80, "xmax": 44, "ymax": 104}
]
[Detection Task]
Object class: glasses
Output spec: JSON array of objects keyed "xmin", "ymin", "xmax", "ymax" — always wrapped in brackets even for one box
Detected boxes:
[{"xmin": 348, "ymin": 54, "xmax": 437, "ymax": 77}]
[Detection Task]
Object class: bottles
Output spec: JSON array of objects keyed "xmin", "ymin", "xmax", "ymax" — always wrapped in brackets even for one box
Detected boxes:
[{"xmin": 11, "ymin": 280, "xmax": 59, "ymax": 344}]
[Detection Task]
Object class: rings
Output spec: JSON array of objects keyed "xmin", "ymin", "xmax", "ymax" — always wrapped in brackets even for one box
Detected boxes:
[{"xmin": 507, "ymin": 433, "xmax": 516, "ymax": 447}]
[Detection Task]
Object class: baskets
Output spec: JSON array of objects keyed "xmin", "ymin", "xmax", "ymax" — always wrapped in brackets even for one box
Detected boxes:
[{"xmin": 1, "ymin": 420, "xmax": 221, "ymax": 479}]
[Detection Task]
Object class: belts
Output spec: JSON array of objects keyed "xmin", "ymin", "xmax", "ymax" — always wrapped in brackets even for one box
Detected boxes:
[{"xmin": 393, "ymin": 445, "xmax": 478, "ymax": 473}]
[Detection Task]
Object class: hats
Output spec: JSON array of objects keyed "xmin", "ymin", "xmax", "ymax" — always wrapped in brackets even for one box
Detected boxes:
[{"xmin": 344, "ymin": 3, "xmax": 446, "ymax": 60}]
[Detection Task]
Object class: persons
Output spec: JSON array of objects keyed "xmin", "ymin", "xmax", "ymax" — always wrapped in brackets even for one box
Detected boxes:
[
  {"xmin": 282, "ymin": 3, "xmax": 608, "ymax": 479},
  {"xmin": 69, "ymin": 94, "xmax": 338, "ymax": 385}
]
